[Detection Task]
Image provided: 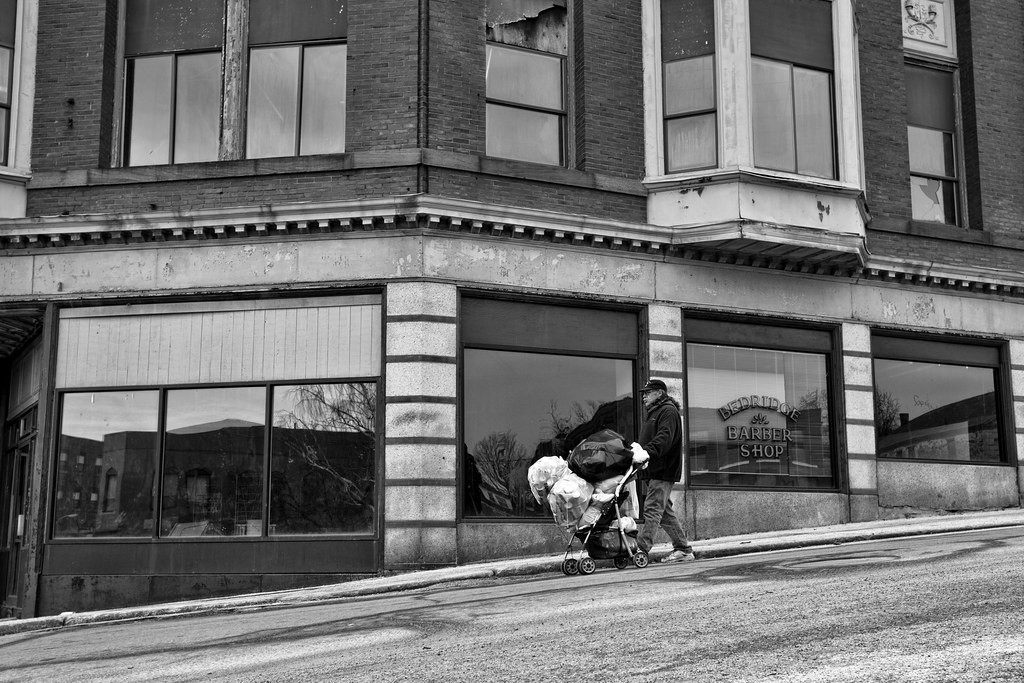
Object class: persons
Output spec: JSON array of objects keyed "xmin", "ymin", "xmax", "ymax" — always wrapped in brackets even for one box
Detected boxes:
[{"xmin": 630, "ymin": 379, "xmax": 695, "ymax": 564}]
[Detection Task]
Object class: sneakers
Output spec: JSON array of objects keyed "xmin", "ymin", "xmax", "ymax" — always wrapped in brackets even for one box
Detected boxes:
[{"xmin": 661, "ymin": 550, "xmax": 695, "ymax": 562}]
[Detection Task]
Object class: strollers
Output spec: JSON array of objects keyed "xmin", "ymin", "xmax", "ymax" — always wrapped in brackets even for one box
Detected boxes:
[{"xmin": 561, "ymin": 459, "xmax": 653, "ymax": 574}]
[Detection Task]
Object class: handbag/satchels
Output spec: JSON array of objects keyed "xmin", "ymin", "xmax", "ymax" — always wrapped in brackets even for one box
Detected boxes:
[{"xmin": 527, "ymin": 429, "xmax": 634, "ymax": 530}]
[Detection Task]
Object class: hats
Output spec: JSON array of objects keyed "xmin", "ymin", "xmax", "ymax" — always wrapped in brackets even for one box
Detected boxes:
[{"xmin": 639, "ymin": 380, "xmax": 666, "ymax": 391}]
